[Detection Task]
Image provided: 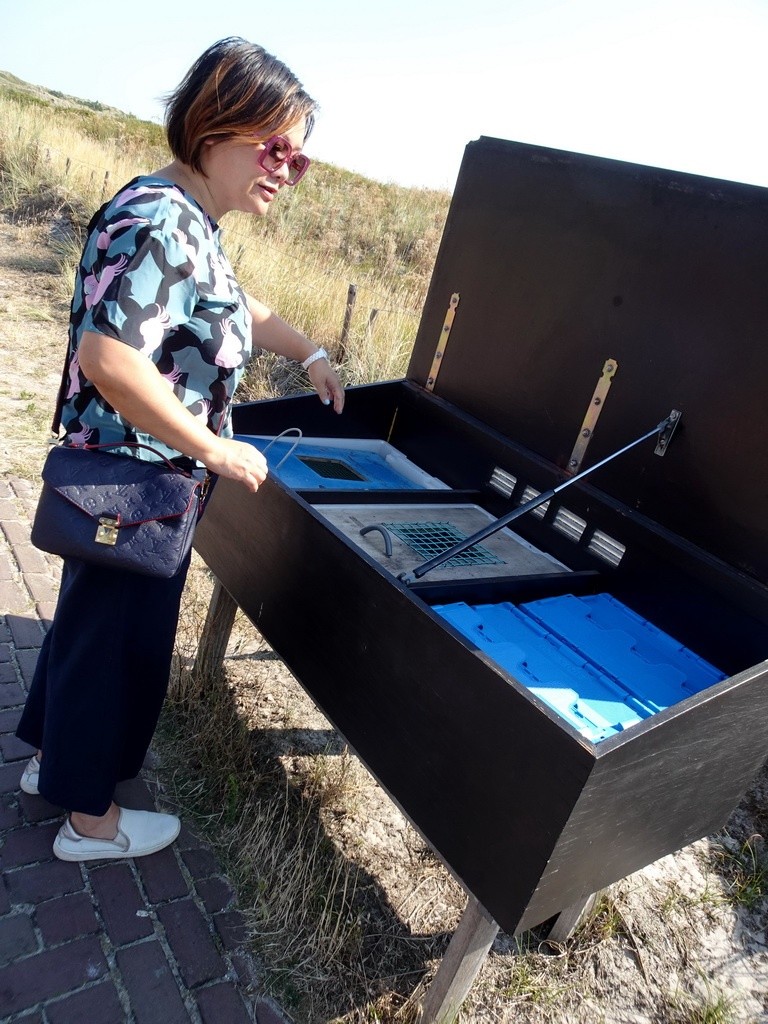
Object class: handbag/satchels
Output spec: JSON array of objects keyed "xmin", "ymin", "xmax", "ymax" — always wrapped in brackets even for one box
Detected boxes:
[{"xmin": 30, "ymin": 441, "xmax": 205, "ymax": 579}]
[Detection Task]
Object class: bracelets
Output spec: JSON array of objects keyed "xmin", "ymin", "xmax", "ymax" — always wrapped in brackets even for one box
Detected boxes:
[{"xmin": 302, "ymin": 347, "xmax": 327, "ymax": 370}]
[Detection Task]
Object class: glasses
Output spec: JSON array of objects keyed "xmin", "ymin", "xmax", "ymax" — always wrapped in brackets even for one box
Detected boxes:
[{"xmin": 252, "ymin": 133, "xmax": 310, "ymax": 186}]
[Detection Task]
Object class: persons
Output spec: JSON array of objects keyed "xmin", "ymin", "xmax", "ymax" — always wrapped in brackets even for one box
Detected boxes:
[{"xmin": 14, "ymin": 35, "xmax": 344, "ymax": 862}]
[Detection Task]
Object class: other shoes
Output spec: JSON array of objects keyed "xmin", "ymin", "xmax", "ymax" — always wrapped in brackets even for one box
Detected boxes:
[
  {"xmin": 52, "ymin": 805, "xmax": 182, "ymax": 863},
  {"xmin": 19, "ymin": 755, "xmax": 42, "ymax": 795}
]
